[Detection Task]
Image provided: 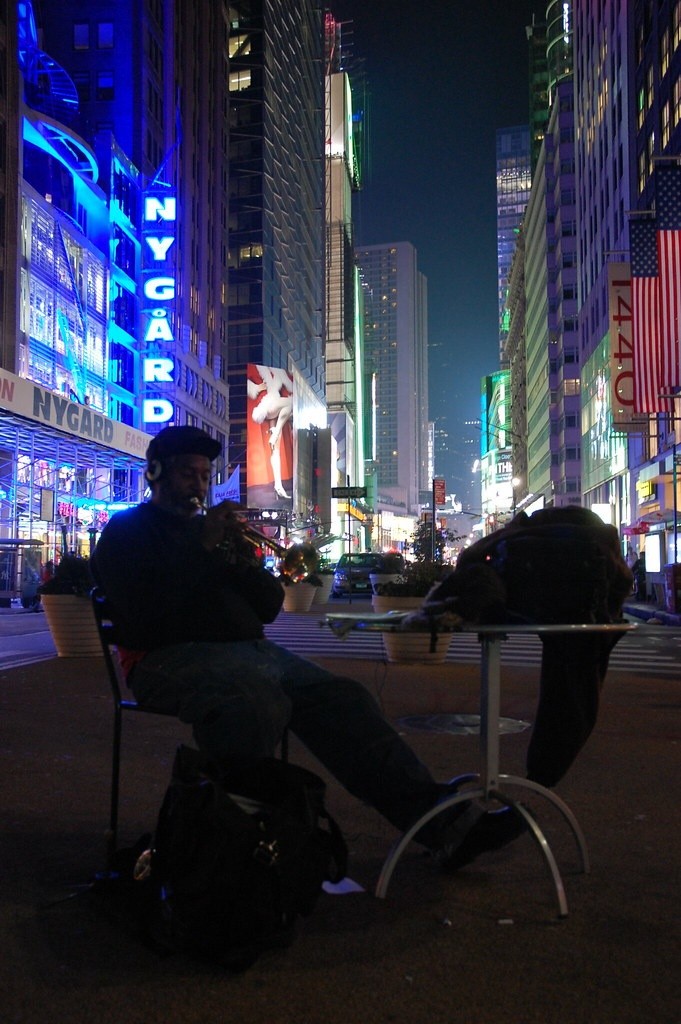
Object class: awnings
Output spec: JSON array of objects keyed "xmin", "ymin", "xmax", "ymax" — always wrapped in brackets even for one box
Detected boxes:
[{"xmin": 623, "ymin": 508, "xmax": 681, "ymax": 535}]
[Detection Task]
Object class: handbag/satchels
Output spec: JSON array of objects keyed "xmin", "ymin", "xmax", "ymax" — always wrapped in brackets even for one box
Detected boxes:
[{"xmin": 148, "ymin": 743, "xmax": 350, "ymax": 976}]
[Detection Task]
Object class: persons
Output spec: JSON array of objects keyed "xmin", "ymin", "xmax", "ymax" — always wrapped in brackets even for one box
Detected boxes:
[
  {"xmin": 92, "ymin": 426, "xmax": 534, "ymax": 886},
  {"xmin": 625, "ymin": 547, "xmax": 645, "ymax": 599},
  {"xmin": 365, "ymin": 548, "xmax": 372, "ymax": 560},
  {"xmin": 252, "ymin": 365, "xmax": 292, "ymax": 500},
  {"xmin": 42, "ymin": 561, "xmax": 53, "ymax": 584}
]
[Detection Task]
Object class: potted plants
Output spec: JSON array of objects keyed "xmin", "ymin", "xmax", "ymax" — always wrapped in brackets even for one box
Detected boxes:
[
  {"xmin": 369, "ymin": 556, "xmax": 458, "ymax": 664},
  {"xmin": 36, "ymin": 556, "xmax": 112, "ymax": 657},
  {"xmin": 277, "ymin": 557, "xmax": 334, "ymax": 613}
]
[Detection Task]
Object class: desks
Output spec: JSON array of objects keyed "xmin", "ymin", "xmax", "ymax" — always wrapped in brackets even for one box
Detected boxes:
[{"xmin": 322, "ymin": 612, "xmax": 641, "ymax": 917}]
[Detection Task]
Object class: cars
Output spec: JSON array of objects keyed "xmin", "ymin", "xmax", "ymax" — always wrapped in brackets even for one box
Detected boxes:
[{"xmin": 331, "ymin": 553, "xmax": 382, "ymax": 596}]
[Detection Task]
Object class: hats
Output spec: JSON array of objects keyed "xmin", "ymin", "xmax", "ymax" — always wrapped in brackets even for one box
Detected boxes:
[{"xmin": 147, "ymin": 426, "xmax": 222, "ymax": 468}]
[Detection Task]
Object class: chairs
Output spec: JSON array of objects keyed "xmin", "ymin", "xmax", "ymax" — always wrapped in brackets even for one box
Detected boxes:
[{"xmin": 88, "ymin": 585, "xmax": 287, "ymax": 870}]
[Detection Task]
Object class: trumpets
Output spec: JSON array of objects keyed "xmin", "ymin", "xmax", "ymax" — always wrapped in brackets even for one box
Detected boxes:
[{"xmin": 191, "ymin": 497, "xmax": 317, "ymax": 583}]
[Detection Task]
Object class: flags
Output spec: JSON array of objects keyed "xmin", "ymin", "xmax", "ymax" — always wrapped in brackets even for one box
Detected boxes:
[{"xmin": 630, "ymin": 163, "xmax": 681, "ymax": 415}]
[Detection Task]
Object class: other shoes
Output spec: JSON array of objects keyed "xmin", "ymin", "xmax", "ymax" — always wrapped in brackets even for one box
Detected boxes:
[{"xmin": 439, "ymin": 802, "xmax": 534, "ymax": 872}]
